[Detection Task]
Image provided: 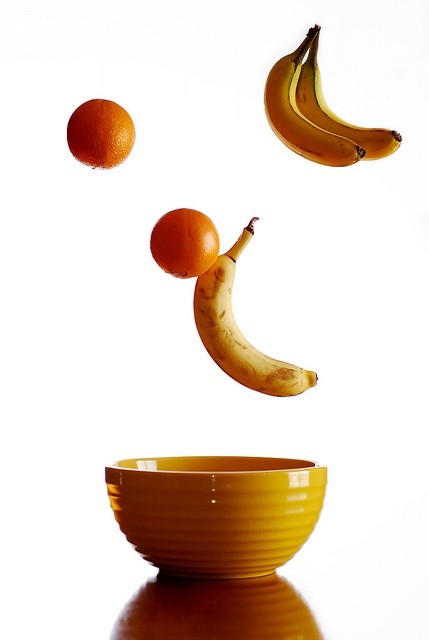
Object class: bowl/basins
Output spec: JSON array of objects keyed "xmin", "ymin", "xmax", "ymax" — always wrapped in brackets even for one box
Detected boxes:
[{"xmin": 104, "ymin": 455, "xmax": 329, "ymax": 580}]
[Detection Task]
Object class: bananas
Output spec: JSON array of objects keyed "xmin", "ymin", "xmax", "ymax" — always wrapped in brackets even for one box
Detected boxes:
[
  {"xmin": 264, "ymin": 25, "xmax": 402, "ymax": 167},
  {"xmin": 194, "ymin": 216, "xmax": 319, "ymax": 397}
]
